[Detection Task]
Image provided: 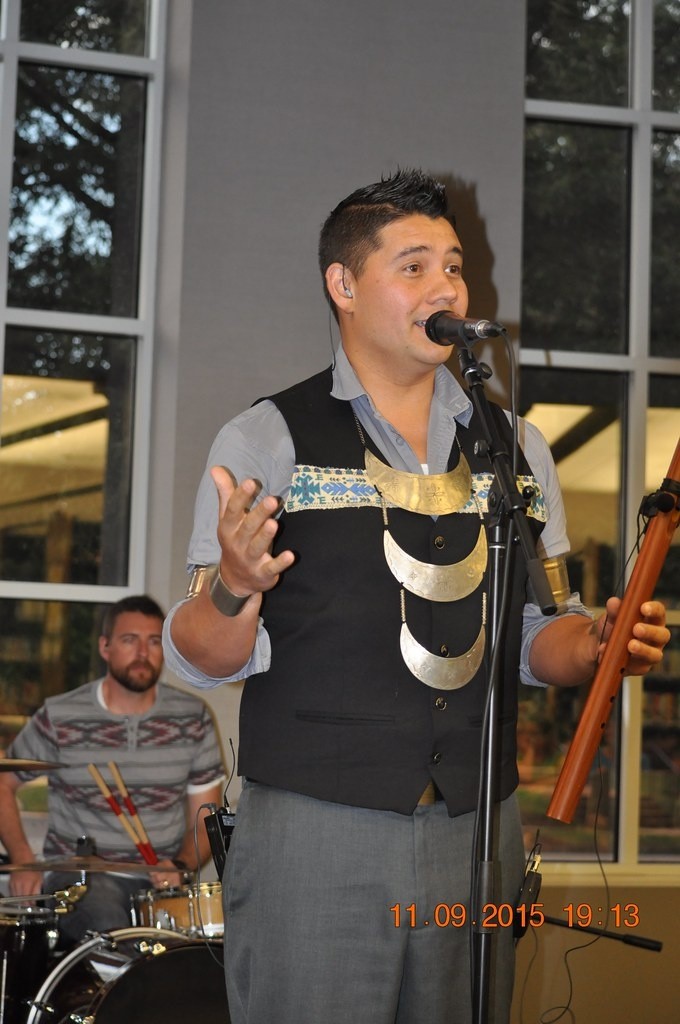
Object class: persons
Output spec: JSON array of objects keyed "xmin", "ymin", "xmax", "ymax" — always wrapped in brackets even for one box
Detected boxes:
[
  {"xmin": 0, "ymin": 595, "xmax": 228, "ymax": 956},
  {"xmin": 160, "ymin": 171, "xmax": 671, "ymax": 1024}
]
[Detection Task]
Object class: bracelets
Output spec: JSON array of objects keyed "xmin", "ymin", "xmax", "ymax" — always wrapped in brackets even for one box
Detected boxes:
[{"xmin": 208, "ymin": 564, "xmax": 252, "ymax": 617}]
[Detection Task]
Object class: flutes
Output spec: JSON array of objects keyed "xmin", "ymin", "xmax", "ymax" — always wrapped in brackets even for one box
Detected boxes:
[{"xmin": 546, "ymin": 437, "xmax": 680, "ymax": 827}]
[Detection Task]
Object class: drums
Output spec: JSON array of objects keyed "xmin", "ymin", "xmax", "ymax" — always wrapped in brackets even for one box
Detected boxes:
[
  {"xmin": 28, "ymin": 924, "xmax": 234, "ymax": 1024},
  {"xmin": 129, "ymin": 879, "xmax": 223, "ymax": 943}
]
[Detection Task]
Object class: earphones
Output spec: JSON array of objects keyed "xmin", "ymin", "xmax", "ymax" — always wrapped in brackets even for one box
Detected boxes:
[{"xmin": 343, "ymin": 287, "xmax": 353, "ymax": 297}]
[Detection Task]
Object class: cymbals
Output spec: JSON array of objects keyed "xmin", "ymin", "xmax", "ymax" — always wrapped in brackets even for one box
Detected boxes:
[
  {"xmin": 0, "ymin": 758, "xmax": 72, "ymax": 773},
  {"xmin": 6, "ymin": 854, "xmax": 188, "ymax": 874}
]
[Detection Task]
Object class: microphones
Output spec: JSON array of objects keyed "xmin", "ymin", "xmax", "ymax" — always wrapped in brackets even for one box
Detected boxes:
[{"xmin": 424, "ymin": 309, "xmax": 507, "ymax": 346}]
